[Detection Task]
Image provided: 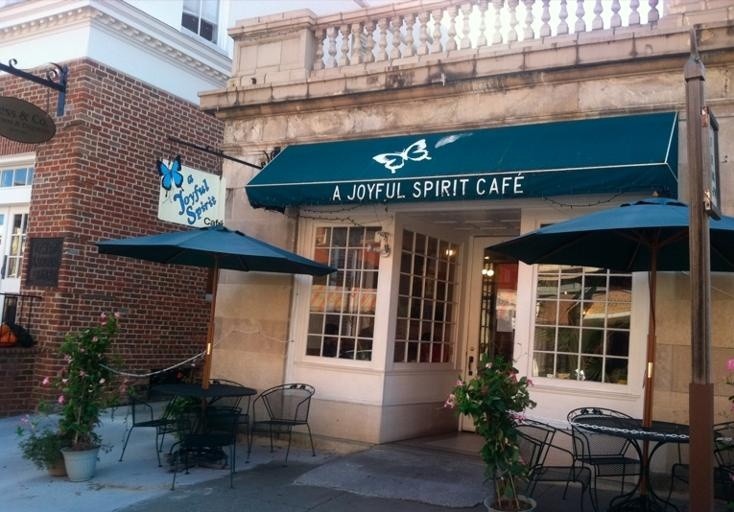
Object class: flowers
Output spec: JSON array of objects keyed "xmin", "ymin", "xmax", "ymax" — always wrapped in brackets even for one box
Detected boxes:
[
  {"xmin": 444, "ymin": 351, "xmax": 539, "ymax": 508},
  {"xmin": 38, "ymin": 308, "xmax": 120, "ymax": 448}
]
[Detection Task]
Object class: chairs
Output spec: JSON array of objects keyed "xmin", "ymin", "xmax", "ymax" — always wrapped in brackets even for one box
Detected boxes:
[
  {"xmin": 514, "ymin": 405, "xmax": 733, "ymax": 512},
  {"xmin": 116, "ymin": 366, "xmax": 315, "ymax": 490}
]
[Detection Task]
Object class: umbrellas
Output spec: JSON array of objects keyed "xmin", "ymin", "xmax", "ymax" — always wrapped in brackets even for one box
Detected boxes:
[
  {"xmin": 486, "ymin": 197, "xmax": 734, "ymax": 429},
  {"xmin": 94, "ymin": 224, "xmax": 338, "ymax": 390}
]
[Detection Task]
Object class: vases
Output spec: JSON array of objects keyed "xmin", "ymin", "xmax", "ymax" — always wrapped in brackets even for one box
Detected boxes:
[{"xmin": 58, "ymin": 446, "xmax": 98, "ymax": 482}]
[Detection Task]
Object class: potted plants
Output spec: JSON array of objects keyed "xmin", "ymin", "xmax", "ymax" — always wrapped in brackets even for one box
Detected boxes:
[{"xmin": 13, "ymin": 416, "xmax": 67, "ymax": 476}]
